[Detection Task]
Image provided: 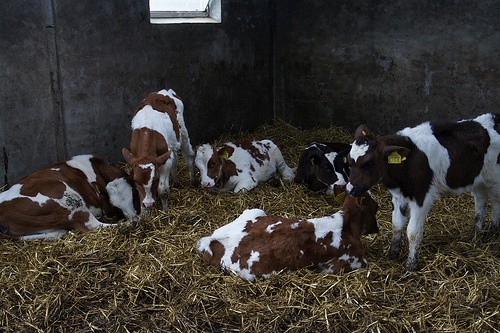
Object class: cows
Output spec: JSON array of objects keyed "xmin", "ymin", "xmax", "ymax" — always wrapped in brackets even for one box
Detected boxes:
[
  {"xmin": 195, "ymin": 139, "xmax": 295, "ymax": 192},
  {"xmin": 346, "ymin": 113, "xmax": 500, "ymax": 272},
  {"xmin": 123, "ymin": 88, "xmax": 194, "ymax": 210},
  {"xmin": 293, "ymin": 140, "xmax": 355, "ymax": 195},
  {"xmin": 0, "ymin": 154, "xmax": 140, "ymax": 241},
  {"xmin": 199, "ymin": 196, "xmax": 378, "ymax": 281}
]
